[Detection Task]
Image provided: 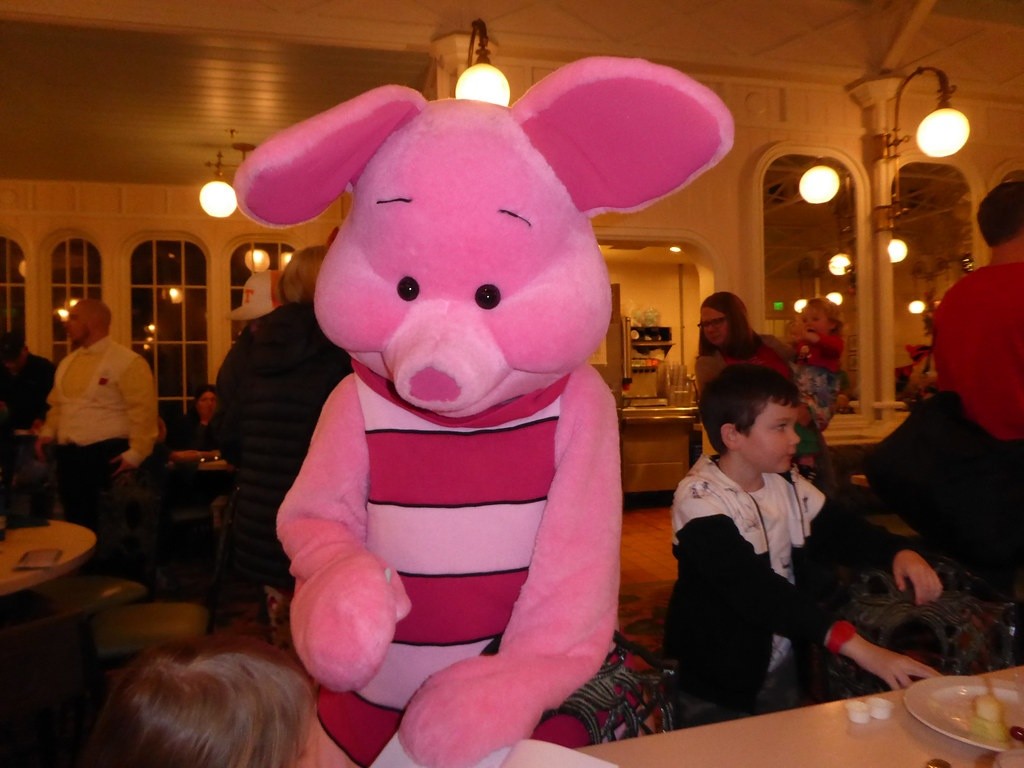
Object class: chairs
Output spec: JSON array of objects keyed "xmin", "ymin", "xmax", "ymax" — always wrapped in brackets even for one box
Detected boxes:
[
  {"xmin": 823, "ymin": 553, "xmax": 1016, "ymax": 701},
  {"xmin": 531, "ymin": 630, "xmax": 678, "ymax": 744}
]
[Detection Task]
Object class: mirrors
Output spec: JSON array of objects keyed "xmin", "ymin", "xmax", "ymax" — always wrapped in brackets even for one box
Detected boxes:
[
  {"xmin": 748, "ymin": 141, "xmax": 874, "ymax": 429},
  {"xmin": 873, "ymin": 148, "xmax": 991, "ymax": 422}
]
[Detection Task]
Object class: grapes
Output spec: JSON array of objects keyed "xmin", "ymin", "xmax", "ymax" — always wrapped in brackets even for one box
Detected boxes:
[{"xmin": 1010, "ymin": 726, "xmax": 1024, "ymax": 742}]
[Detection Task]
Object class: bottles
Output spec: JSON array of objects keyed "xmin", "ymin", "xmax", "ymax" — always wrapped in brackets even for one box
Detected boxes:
[{"xmin": 10, "ymin": 435, "xmax": 41, "ymax": 523}]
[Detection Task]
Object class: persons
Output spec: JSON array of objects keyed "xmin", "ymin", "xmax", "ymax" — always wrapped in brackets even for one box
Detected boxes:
[
  {"xmin": 0, "ymin": 243, "xmax": 355, "ymax": 768},
  {"xmin": 657, "ymin": 182, "xmax": 1024, "ymax": 716}
]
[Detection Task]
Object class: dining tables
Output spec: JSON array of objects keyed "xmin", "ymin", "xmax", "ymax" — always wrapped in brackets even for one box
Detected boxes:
[{"xmin": 574, "ymin": 666, "xmax": 1024, "ymax": 768}]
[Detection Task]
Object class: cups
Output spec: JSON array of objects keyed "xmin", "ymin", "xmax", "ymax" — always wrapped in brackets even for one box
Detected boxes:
[
  {"xmin": 866, "ymin": 697, "xmax": 894, "ymax": 719},
  {"xmin": 845, "ymin": 700, "xmax": 870, "ymax": 723}
]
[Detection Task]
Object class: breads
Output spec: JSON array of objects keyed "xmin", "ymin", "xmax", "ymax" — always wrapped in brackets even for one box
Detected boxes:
[{"xmin": 969, "ymin": 694, "xmax": 1012, "ymax": 742}]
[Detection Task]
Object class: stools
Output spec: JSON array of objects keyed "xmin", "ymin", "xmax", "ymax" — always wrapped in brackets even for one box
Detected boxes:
[
  {"xmin": 165, "ymin": 503, "xmax": 213, "ymax": 569},
  {"xmin": 79, "ymin": 602, "xmax": 215, "ymax": 711}
]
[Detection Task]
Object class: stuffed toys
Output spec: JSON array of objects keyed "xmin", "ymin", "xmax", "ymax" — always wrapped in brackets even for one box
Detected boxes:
[{"xmin": 229, "ymin": 57, "xmax": 736, "ymax": 767}]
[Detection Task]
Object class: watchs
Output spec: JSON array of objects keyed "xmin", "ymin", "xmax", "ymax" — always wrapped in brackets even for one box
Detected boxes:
[{"xmin": 826, "ymin": 618, "xmax": 857, "ymax": 655}]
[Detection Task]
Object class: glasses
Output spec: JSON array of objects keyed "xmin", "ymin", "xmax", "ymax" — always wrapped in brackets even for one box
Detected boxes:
[{"xmin": 698, "ymin": 316, "xmax": 726, "ymax": 329}]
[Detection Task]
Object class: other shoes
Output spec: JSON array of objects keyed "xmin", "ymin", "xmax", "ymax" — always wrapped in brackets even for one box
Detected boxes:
[{"xmin": 797, "ymin": 465, "xmax": 823, "ymax": 484}]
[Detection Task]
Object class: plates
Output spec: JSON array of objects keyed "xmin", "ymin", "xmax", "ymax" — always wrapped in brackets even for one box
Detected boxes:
[{"xmin": 903, "ymin": 675, "xmax": 1024, "ymax": 751}]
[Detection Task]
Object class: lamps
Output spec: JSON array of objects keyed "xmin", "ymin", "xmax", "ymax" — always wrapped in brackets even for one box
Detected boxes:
[
  {"xmin": 199, "ymin": 142, "xmax": 256, "ymax": 218},
  {"xmin": 888, "ymin": 65, "xmax": 970, "ymax": 219},
  {"xmin": 455, "ymin": 17, "xmax": 511, "ymax": 106}
]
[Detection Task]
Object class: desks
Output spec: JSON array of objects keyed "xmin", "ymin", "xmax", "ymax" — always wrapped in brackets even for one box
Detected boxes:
[{"xmin": 0, "ymin": 515, "xmax": 97, "ymax": 598}]
